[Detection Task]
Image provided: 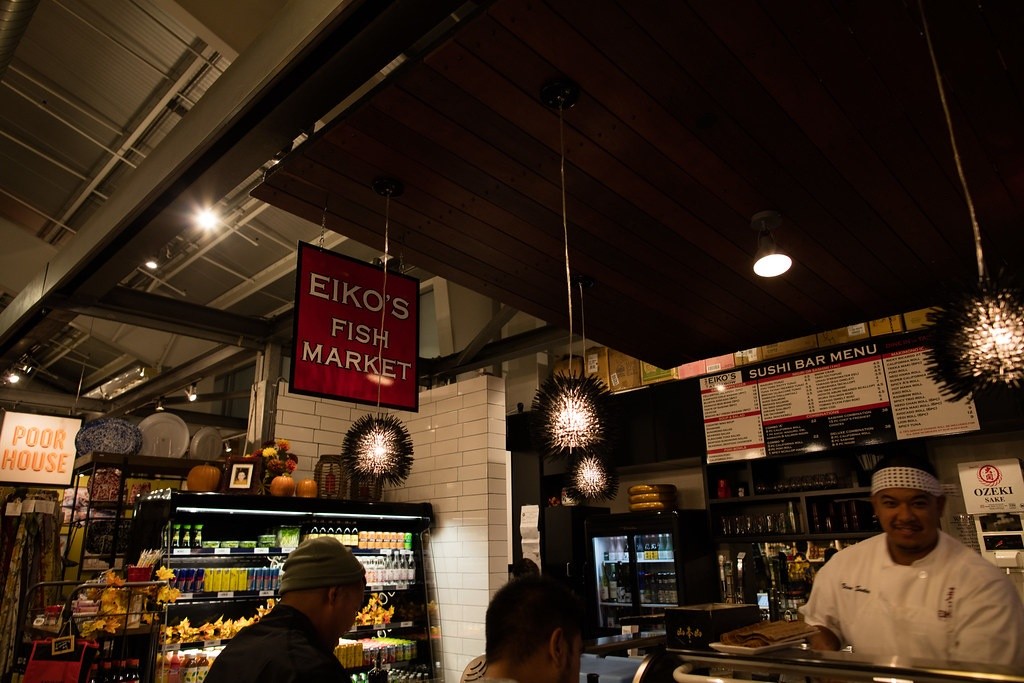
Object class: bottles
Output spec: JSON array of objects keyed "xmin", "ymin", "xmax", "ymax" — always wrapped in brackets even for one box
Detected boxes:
[
  {"xmin": 786, "ymin": 501, "xmax": 802, "ymax": 534},
  {"xmin": 810, "ymin": 498, "xmax": 878, "ymax": 534},
  {"xmin": 350, "ymin": 665, "xmax": 429, "ymax": 683},
  {"xmin": 602, "ymin": 605, "xmax": 667, "ymax": 629},
  {"xmin": 156, "ymin": 646, "xmax": 227, "ymax": 683},
  {"xmin": 352, "ymin": 551, "xmax": 416, "ymax": 589},
  {"xmin": 639, "ymin": 568, "xmax": 678, "ymax": 604},
  {"xmin": 600, "ymin": 562, "xmax": 631, "ymax": 602},
  {"xmin": 89, "ymin": 659, "xmax": 141, "ymax": 683},
  {"xmin": 607, "ymin": 536, "xmax": 630, "ymax": 562},
  {"xmin": 634, "ymin": 533, "xmax": 674, "ymax": 560}
]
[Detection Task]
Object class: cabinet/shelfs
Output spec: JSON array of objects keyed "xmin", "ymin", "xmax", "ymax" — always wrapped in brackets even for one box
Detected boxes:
[
  {"xmin": 124, "ymin": 489, "xmax": 444, "ymax": 683},
  {"xmin": 706, "ymin": 438, "xmax": 899, "ymax": 620}
]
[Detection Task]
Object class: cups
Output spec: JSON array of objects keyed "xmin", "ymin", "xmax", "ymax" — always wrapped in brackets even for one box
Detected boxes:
[
  {"xmin": 756, "ymin": 473, "xmax": 839, "ymax": 494},
  {"xmin": 718, "ymin": 480, "xmax": 731, "ymax": 497},
  {"xmin": 717, "ymin": 512, "xmax": 791, "ymax": 533}
]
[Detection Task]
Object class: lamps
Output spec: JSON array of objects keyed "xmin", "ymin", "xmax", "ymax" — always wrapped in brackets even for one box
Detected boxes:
[
  {"xmin": 525, "ymin": 72, "xmax": 607, "ymax": 451},
  {"xmin": 569, "ymin": 276, "xmax": 619, "ymax": 499},
  {"xmin": 915, "ymin": 8, "xmax": 1024, "ymax": 404},
  {"xmin": 341, "ymin": 168, "xmax": 415, "ymax": 485},
  {"xmin": 749, "ymin": 227, "xmax": 793, "ymax": 278}
]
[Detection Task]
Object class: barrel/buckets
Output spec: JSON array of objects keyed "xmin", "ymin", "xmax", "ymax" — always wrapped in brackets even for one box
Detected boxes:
[{"xmin": 127, "ymin": 567, "xmax": 152, "ymax": 582}]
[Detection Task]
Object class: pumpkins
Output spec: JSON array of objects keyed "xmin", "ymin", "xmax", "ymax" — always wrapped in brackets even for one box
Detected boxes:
[
  {"xmin": 187, "ymin": 462, "xmax": 220, "ymax": 492},
  {"xmin": 297, "ymin": 477, "xmax": 318, "ymax": 498},
  {"xmin": 271, "ymin": 473, "xmax": 294, "ymax": 497}
]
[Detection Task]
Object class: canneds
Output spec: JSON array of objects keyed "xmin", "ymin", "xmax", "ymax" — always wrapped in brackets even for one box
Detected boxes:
[
  {"xmin": 170, "ymin": 567, "xmax": 279, "ymax": 593},
  {"xmin": 358, "ymin": 531, "xmax": 412, "ymax": 550},
  {"xmin": 334, "ymin": 638, "xmax": 417, "ymax": 667}
]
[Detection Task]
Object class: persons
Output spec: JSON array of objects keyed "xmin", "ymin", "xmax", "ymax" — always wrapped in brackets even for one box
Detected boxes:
[
  {"xmin": 203, "ymin": 536, "xmax": 366, "ymax": 683},
  {"xmin": 475, "ymin": 575, "xmax": 598, "ymax": 683},
  {"xmin": 234, "ymin": 471, "xmax": 247, "ymax": 484},
  {"xmin": 797, "ymin": 449, "xmax": 1024, "ymax": 672}
]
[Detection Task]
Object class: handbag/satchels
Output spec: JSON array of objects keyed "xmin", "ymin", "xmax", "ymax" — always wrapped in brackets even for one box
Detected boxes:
[{"xmin": 23, "ymin": 621, "xmax": 99, "ymax": 683}]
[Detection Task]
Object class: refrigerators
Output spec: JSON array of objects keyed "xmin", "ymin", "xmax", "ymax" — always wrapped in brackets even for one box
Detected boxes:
[{"xmin": 578, "ymin": 508, "xmax": 715, "ymax": 632}]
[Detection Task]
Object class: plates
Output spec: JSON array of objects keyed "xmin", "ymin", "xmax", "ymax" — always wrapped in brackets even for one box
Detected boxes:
[
  {"xmin": 74, "ymin": 417, "xmax": 143, "ymax": 456},
  {"xmin": 86, "ymin": 468, "xmax": 122, "ymax": 502},
  {"xmin": 189, "ymin": 426, "xmax": 223, "ymax": 460},
  {"xmin": 708, "ymin": 637, "xmax": 806, "ymax": 656},
  {"xmin": 136, "ymin": 412, "xmax": 190, "ymax": 458}
]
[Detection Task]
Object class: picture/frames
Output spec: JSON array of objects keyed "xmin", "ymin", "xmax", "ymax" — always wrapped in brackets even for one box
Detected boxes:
[{"xmin": 221, "ymin": 455, "xmax": 263, "ymax": 493}]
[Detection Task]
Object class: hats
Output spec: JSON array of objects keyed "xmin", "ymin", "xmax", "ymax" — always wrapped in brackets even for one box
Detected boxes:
[{"xmin": 278, "ymin": 537, "xmax": 366, "ymax": 594}]
